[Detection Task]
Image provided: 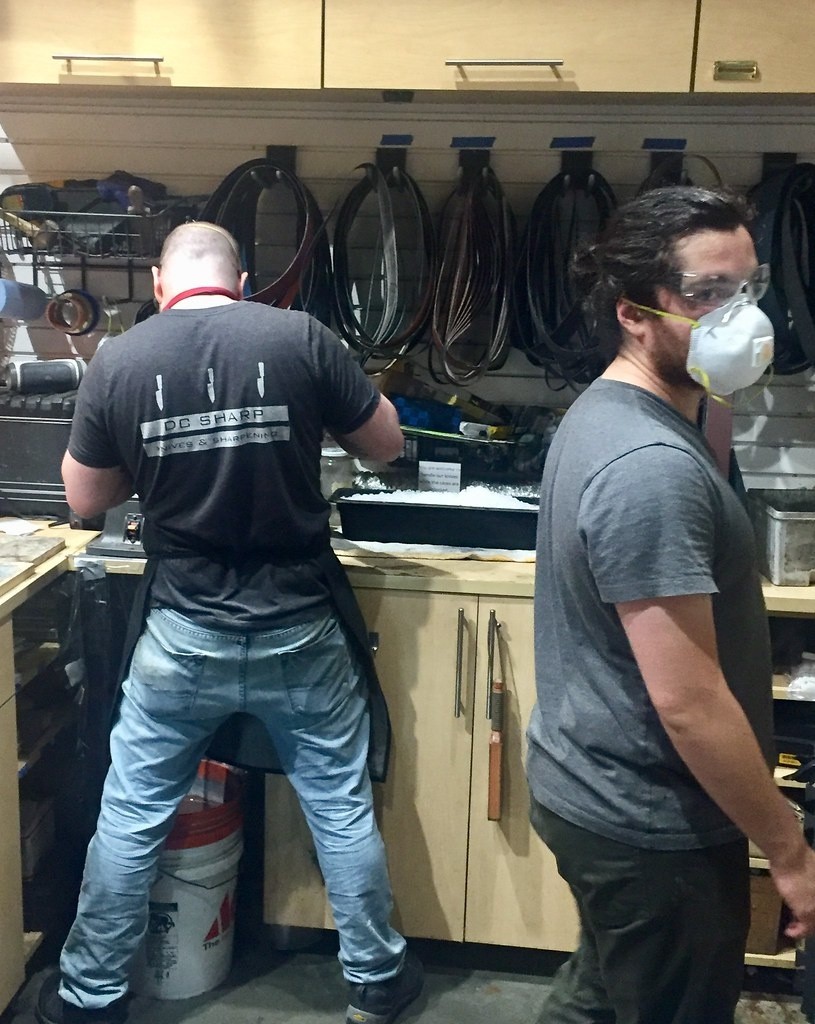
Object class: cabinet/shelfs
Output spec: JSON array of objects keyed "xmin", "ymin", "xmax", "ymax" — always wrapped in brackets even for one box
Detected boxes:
[
  {"xmin": 0, "ymin": 572, "xmax": 815, "ymax": 1017},
  {"xmin": 1, "ymin": 1, "xmax": 815, "ymax": 106}
]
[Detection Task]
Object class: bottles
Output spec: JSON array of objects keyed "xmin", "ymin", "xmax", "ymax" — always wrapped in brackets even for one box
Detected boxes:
[{"xmin": 320, "ymin": 448, "xmax": 352, "ymax": 527}]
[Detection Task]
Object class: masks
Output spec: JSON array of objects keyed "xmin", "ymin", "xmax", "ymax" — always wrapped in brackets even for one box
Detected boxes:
[{"xmin": 626, "ymin": 299, "xmax": 779, "ymax": 398}]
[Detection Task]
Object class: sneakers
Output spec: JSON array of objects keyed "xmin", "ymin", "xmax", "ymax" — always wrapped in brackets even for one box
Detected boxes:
[
  {"xmin": 343, "ymin": 956, "xmax": 426, "ymax": 1024},
  {"xmin": 37, "ymin": 971, "xmax": 136, "ymax": 1024}
]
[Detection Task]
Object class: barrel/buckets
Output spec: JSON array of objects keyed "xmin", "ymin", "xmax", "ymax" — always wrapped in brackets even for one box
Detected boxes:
[{"xmin": 127, "ymin": 759, "xmax": 244, "ymax": 1000}]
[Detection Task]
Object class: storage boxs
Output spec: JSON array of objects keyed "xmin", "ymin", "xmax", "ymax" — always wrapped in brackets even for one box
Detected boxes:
[
  {"xmin": 0, "ymin": 391, "xmax": 74, "ymax": 525},
  {"xmin": 747, "ymin": 489, "xmax": 815, "ymax": 588},
  {"xmin": 745, "ymin": 872, "xmax": 783, "ymax": 956},
  {"xmin": 328, "ymin": 487, "xmax": 540, "ymax": 553},
  {"xmin": 390, "ymin": 425, "xmax": 519, "ymax": 472}
]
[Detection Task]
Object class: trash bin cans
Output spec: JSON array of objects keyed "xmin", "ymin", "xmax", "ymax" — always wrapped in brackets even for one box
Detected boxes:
[{"xmin": 133, "ymin": 759, "xmax": 246, "ymax": 1000}]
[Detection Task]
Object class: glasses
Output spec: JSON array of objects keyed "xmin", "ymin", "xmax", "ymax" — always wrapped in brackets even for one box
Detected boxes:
[{"xmin": 655, "ymin": 264, "xmax": 772, "ymax": 309}]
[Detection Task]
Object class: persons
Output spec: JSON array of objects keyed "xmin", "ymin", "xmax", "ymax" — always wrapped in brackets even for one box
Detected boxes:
[
  {"xmin": 36, "ymin": 221, "xmax": 424, "ymax": 1024},
  {"xmin": 525, "ymin": 185, "xmax": 815, "ymax": 1024}
]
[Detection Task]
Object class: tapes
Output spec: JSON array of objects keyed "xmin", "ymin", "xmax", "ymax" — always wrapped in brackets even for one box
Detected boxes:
[{"xmin": 46, "ymin": 289, "xmax": 100, "ymax": 336}]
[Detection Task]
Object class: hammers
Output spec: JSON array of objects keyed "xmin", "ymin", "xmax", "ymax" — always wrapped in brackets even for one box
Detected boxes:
[{"xmin": 0, "ymin": 205, "xmax": 60, "ymax": 251}]
